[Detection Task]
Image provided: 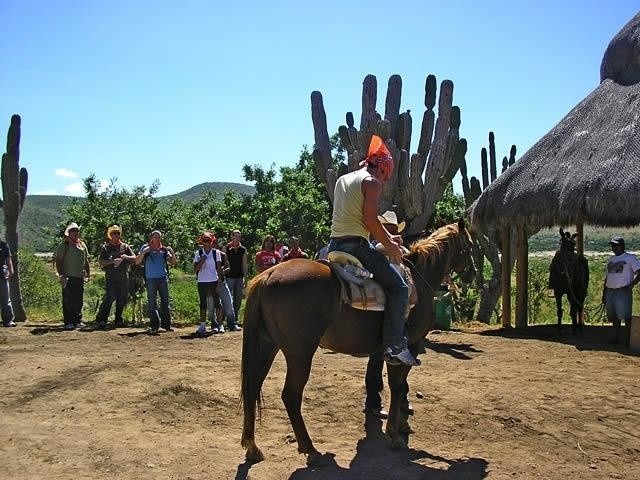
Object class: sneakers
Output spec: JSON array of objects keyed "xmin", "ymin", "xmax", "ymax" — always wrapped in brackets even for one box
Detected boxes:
[
  {"xmin": 211, "ymin": 326, "xmax": 218, "ymax": 333},
  {"xmin": 219, "ymin": 325, "xmax": 224, "ymax": 333},
  {"xmin": 74, "ymin": 322, "xmax": 86, "ymax": 328},
  {"xmin": 231, "ymin": 324, "xmax": 241, "ymax": 331},
  {"xmin": 196, "ymin": 326, "xmax": 207, "ymax": 333},
  {"xmin": 3, "ymin": 322, "xmax": 17, "ymax": 327},
  {"xmin": 65, "ymin": 323, "xmax": 74, "ymax": 330},
  {"xmin": 384, "ymin": 345, "xmax": 419, "ymax": 366}
]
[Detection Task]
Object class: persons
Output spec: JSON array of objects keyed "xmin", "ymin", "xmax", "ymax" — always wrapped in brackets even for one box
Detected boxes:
[
  {"xmin": 326, "ymin": 133, "xmax": 421, "ymax": 369},
  {"xmin": 363, "ymin": 210, "xmax": 414, "ymax": 420},
  {"xmin": 55, "ymin": 222, "xmax": 91, "ymax": 330},
  {"xmin": 255, "ymin": 234, "xmax": 282, "ymax": 274},
  {"xmin": 281, "ymin": 235, "xmax": 309, "ymax": 263},
  {"xmin": 93, "ymin": 224, "xmax": 137, "ymax": 328},
  {"xmin": 197, "ymin": 232, "xmax": 242, "ymax": 333},
  {"xmin": 220, "ymin": 229, "xmax": 249, "ymax": 327},
  {"xmin": 135, "ymin": 229, "xmax": 177, "ymax": 333},
  {"xmin": 602, "ymin": 236, "xmax": 640, "ymax": 350},
  {"xmin": 0, "ymin": 238, "xmax": 17, "ymax": 328},
  {"xmin": 276, "ymin": 237, "xmax": 289, "ymax": 257},
  {"xmin": 193, "ymin": 231, "xmax": 226, "ymax": 334}
]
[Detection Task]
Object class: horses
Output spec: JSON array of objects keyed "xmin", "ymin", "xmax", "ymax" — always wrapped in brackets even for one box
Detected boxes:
[
  {"xmin": 238, "ymin": 215, "xmax": 480, "ymax": 468},
  {"xmin": 547, "ymin": 227, "xmax": 591, "ymax": 339}
]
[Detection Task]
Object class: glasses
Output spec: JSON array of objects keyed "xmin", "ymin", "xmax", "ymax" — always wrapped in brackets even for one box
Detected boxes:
[{"xmin": 110, "ymin": 232, "xmax": 120, "ymax": 235}]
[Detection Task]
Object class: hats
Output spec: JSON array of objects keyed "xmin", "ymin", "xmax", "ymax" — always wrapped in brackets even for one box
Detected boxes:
[
  {"xmin": 608, "ymin": 236, "xmax": 625, "ymax": 246},
  {"xmin": 64, "ymin": 223, "xmax": 79, "ymax": 236},
  {"xmin": 198, "ymin": 232, "xmax": 217, "ymax": 245},
  {"xmin": 377, "ymin": 210, "xmax": 406, "ymax": 232},
  {"xmin": 107, "ymin": 225, "xmax": 120, "ymax": 240}
]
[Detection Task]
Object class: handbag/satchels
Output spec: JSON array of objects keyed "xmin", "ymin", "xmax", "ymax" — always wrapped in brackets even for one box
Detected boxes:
[{"xmin": 1, "ymin": 265, "xmax": 12, "ymax": 279}]
